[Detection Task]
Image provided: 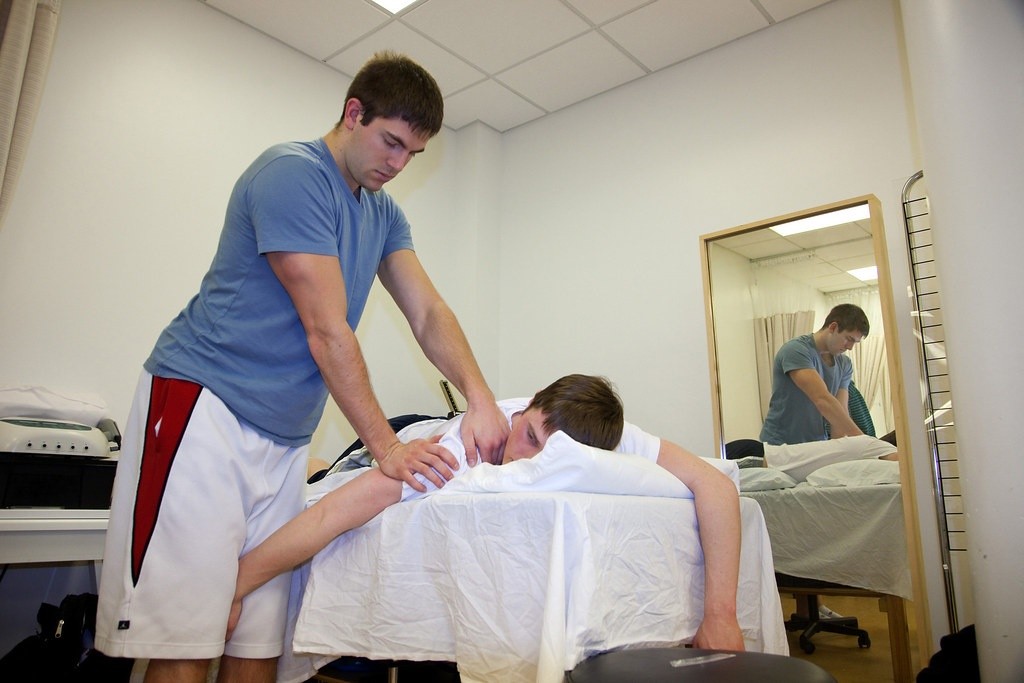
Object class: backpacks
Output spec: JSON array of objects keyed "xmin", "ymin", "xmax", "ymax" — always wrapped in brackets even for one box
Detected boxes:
[{"xmin": 0, "ymin": 592, "xmax": 135, "ymax": 683}]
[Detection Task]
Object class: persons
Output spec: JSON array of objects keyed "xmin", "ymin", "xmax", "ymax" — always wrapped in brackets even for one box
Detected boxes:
[
  {"xmin": 759, "ymin": 304, "xmax": 870, "ymax": 621},
  {"xmin": 724, "ymin": 430, "xmax": 901, "ymax": 483},
  {"xmin": 94, "ymin": 50, "xmax": 510, "ymax": 683},
  {"xmin": 224, "ymin": 373, "xmax": 746, "ymax": 650}
]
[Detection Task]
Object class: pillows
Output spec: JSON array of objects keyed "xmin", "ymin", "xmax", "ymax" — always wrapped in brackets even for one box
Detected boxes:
[
  {"xmin": 739, "ymin": 467, "xmax": 798, "ymax": 492},
  {"xmin": 416, "ymin": 430, "xmax": 740, "ymax": 497},
  {"xmin": 805, "ymin": 459, "xmax": 899, "ymax": 486}
]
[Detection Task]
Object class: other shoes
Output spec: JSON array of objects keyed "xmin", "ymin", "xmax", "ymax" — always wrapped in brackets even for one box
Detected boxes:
[{"xmin": 819, "ymin": 604, "xmax": 844, "ymax": 620}]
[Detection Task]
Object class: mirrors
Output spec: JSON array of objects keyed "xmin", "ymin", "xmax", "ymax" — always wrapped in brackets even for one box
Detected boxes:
[{"xmin": 699, "ymin": 195, "xmax": 934, "ymax": 683}]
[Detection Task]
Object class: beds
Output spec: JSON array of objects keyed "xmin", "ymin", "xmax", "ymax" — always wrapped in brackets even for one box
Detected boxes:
[
  {"xmin": 290, "ymin": 496, "xmax": 788, "ymax": 683},
  {"xmin": 740, "ymin": 484, "xmax": 913, "ymax": 683}
]
